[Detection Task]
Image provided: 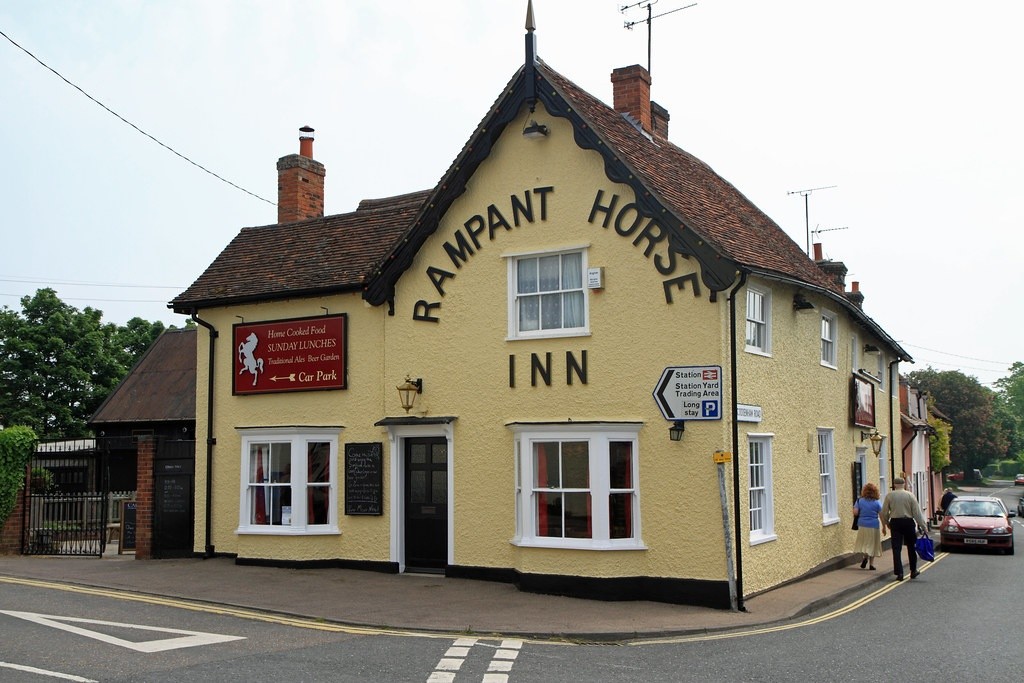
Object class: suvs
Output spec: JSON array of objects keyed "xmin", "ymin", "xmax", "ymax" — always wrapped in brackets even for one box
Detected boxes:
[{"xmin": 947, "ymin": 469, "xmax": 984, "ymax": 481}]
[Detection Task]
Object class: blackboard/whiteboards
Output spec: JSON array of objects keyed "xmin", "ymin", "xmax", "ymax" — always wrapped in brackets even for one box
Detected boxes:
[
  {"xmin": 344, "ymin": 441, "xmax": 384, "ymax": 516},
  {"xmin": 117, "ymin": 499, "xmax": 136, "ymax": 555}
]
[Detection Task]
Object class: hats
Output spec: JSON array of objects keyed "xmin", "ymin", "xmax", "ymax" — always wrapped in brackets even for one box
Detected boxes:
[
  {"xmin": 946, "ymin": 487, "xmax": 953, "ymax": 492},
  {"xmin": 893, "ymin": 478, "xmax": 905, "ymax": 484}
]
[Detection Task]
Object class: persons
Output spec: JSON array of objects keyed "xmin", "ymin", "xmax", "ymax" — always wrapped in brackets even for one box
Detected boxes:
[
  {"xmin": 938, "ymin": 487, "xmax": 958, "ymax": 513},
  {"xmin": 853, "ymin": 483, "xmax": 886, "ymax": 570},
  {"xmin": 882, "ymin": 478, "xmax": 929, "ymax": 581}
]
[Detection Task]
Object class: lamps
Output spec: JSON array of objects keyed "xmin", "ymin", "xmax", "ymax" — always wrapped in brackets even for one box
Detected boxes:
[
  {"xmin": 793, "ymin": 293, "xmax": 818, "ymax": 314},
  {"xmin": 521, "ymin": 98, "xmax": 549, "ymax": 139},
  {"xmin": 669, "ymin": 420, "xmax": 685, "ymax": 441},
  {"xmin": 861, "ymin": 428, "xmax": 883, "ymax": 458},
  {"xmin": 395, "ymin": 374, "xmax": 422, "ymax": 414},
  {"xmin": 864, "ymin": 344, "xmax": 881, "ymax": 356}
]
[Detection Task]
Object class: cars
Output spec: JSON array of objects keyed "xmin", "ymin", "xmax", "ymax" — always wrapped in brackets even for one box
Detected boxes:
[
  {"xmin": 1017, "ymin": 493, "xmax": 1024, "ymax": 517},
  {"xmin": 936, "ymin": 496, "xmax": 1017, "ymax": 555},
  {"xmin": 1015, "ymin": 477, "xmax": 1024, "ymax": 486},
  {"xmin": 1016, "ymin": 474, "xmax": 1024, "ymax": 477}
]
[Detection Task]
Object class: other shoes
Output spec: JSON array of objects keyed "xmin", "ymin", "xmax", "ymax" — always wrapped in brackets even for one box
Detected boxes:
[
  {"xmin": 860, "ymin": 559, "xmax": 868, "ymax": 568},
  {"xmin": 910, "ymin": 569, "xmax": 920, "ymax": 578},
  {"xmin": 896, "ymin": 575, "xmax": 903, "ymax": 581},
  {"xmin": 869, "ymin": 565, "xmax": 876, "ymax": 570}
]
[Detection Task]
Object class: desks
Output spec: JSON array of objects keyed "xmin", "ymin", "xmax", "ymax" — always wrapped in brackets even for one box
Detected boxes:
[{"xmin": 106, "ymin": 523, "xmax": 122, "ymax": 544}]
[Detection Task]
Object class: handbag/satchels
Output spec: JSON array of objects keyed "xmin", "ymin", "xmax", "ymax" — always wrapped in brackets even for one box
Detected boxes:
[
  {"xmin": 851, "ymin": 498, "xmax": 859, "ymax": 530},
  {"xmin": 915, "ymin": 533, "xmax": 934, "ymax": 562}
]
